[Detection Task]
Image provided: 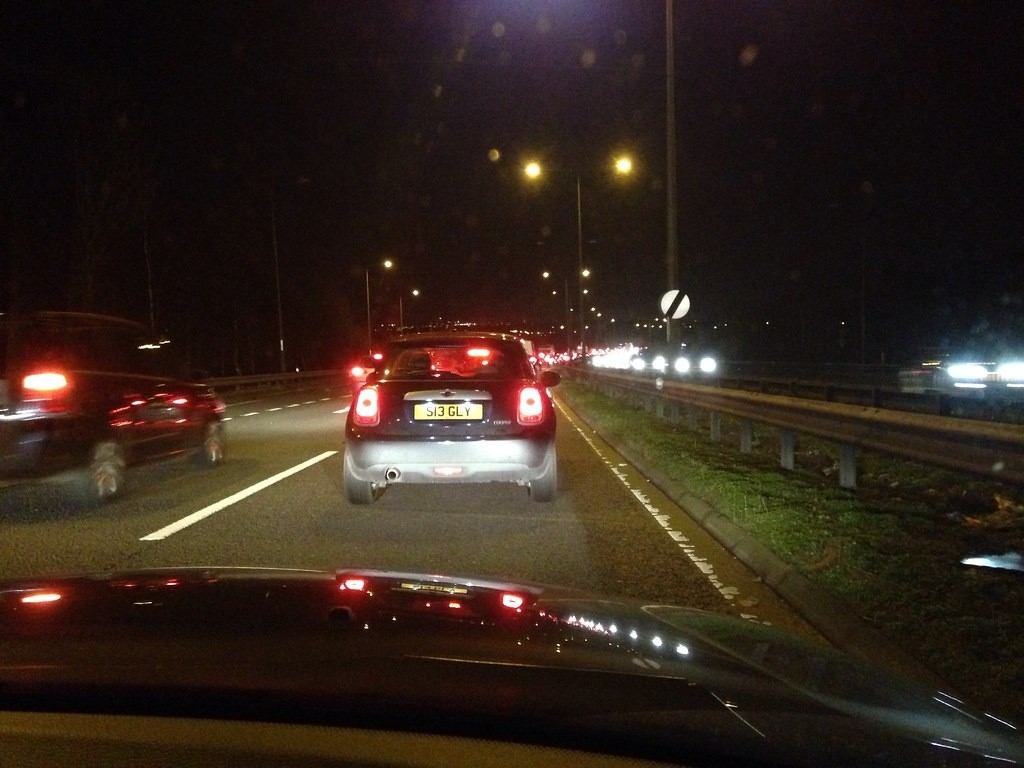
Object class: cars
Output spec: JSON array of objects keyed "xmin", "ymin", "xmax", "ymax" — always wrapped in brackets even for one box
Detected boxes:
[
  {"xmin": 344, "ymin": 333, "xmax": 561, "ymax": 506},
  {"xmin": 352, "ymin": 352, "xmax": 383, "ymax": 394},
  {"xmin": 1, "ymin": 310, "xmax": 226, "ymax": 506},
  {"xmin": 528, "ymin": 343, "xmax": 588, "ymax": 376}
]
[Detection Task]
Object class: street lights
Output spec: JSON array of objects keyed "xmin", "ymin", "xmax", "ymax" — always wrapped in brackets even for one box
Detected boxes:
[{"xmin": 369, "ymin": 142, "xmax": 681, "ymax": 340}]
